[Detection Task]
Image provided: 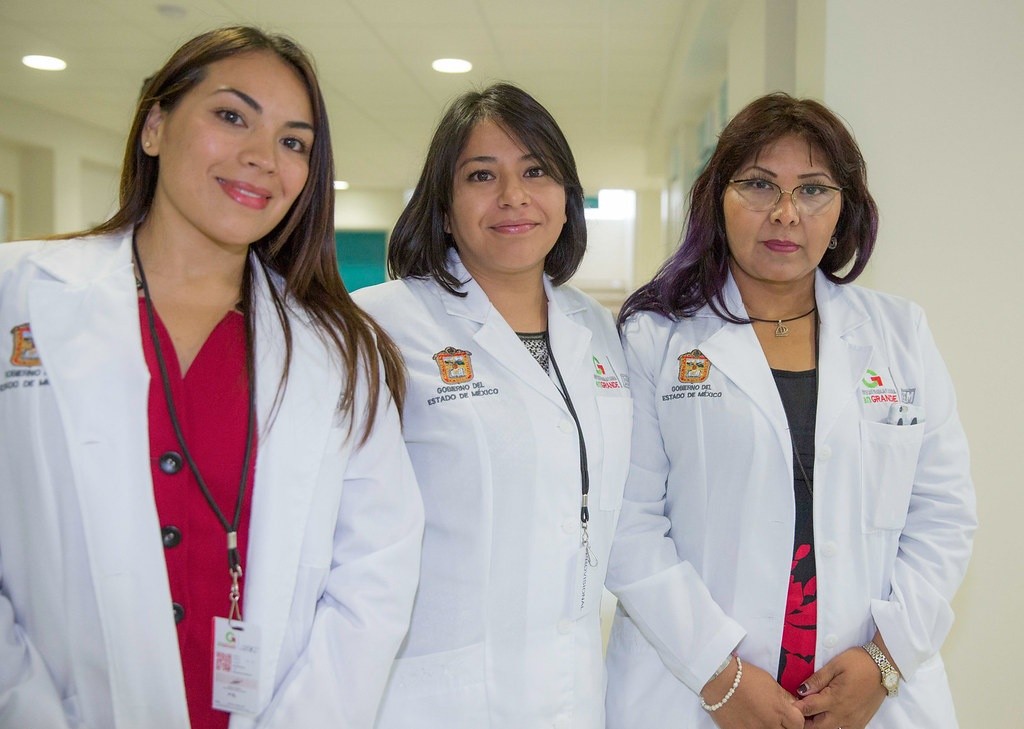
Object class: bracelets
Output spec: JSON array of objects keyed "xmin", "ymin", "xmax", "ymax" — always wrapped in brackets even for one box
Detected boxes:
[{"xmin": 700, "ymin": 651, "xmax": 742, "ymax": 711}]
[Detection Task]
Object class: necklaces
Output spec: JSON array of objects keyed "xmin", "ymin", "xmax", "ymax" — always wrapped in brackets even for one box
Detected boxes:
[{"xmin": 749, "ymin": 307, "xmax": 816, "ymax": 337}]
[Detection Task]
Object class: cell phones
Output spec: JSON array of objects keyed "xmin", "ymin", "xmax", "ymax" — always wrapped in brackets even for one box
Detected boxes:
[{"xmin": 888, "ymin": 403, "xmax": 924, "ymax": 425}]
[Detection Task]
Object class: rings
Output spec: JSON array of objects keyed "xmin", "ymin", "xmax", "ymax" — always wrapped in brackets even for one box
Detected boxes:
[{"xmin": 838, "ymin": 727, "xmax": 842, "ymax": 729}]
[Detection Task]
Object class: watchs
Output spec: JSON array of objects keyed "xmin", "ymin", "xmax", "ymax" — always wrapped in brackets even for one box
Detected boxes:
[{"xmin": 862, "ymin": 642, "xmax": 899, "ymax": 697}]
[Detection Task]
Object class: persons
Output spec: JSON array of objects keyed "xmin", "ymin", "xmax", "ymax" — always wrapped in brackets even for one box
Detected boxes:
[
  {"xmin": 624, "ymin": 97, "xmax": 975, "ymax": 729},
  {"xmin": 0, "ymin": 28, "xmax": 421, "ymax": 729},
  {"xmin": 348, "ymin": 83, "xmax": 630, "ymax": 729}
]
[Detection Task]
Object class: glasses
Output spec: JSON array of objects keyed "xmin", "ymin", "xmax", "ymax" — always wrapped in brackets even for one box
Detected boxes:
[{"xmin": 727, "ymin": 178, "xmax": 845, "ymax": 217}]
